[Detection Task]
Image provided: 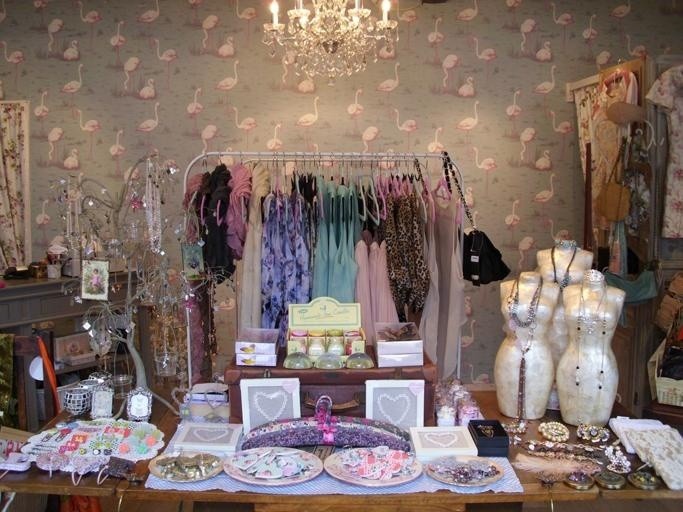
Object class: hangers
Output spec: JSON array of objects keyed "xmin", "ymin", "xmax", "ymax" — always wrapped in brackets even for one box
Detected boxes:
[{"xmin": 187, "ymin": 151, "xmax": 463, "ymax": 227}]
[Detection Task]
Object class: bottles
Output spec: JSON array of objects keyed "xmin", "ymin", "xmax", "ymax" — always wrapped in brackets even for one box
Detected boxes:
[
  {"xmin": 307, "ymin": 329, "xmax": 327, "ymax": 357},
  {"xmin": 345, "ymin": 329, "xmax": 362, "ymax": 355},
  {"xmin": 288, "ymin": 329, "xmax": 309, "ymax": 354},
  {"xmin": 325, "ymin": 328, "xmax": 346, "ymax": 356}
]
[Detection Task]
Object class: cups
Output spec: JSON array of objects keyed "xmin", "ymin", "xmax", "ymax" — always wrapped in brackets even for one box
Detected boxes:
[
  {"xmin": 156, "ymin": 349, "xmax": 178, "ymax": 378},
  {"xmin": 110, "ymin": 373, "xmax": 133, "ymax": 400},
  {"xmin": 47, "ymin": 263, "xmax": 62, "ymax": 279}
]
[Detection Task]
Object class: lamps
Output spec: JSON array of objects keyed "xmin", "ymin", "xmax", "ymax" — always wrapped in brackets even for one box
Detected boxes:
[{"xmin": 262, "ymin": 0, "xmax": 400, "ymax": 85}]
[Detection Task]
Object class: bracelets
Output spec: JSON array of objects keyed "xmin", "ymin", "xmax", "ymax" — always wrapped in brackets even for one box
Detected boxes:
[
  {"xmin": 522, "ymin": 438, "xmax": 631, "ymax": 474},
  {"xmin": 578, "ymin": 421, "xmax": 610, "ymax": 441},
  {"xmin": 538, "ymin": 420, "xmax": 572, "ymax": 441},
  {"xmin": 500, "ymin": 419, "xmax": 526, "ymax": 434}
]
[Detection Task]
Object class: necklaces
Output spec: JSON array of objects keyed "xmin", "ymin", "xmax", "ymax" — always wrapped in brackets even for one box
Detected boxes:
[
  {"xmin": 573, "ymin": 282, "xmax": 607, "ymax": 422},
  {"xmin": 57, "ymin": 149, "xmax": 171, "ymax": 256},
  {"xmin": 549, "ymin": 245, "xmax": 576, "ymax": 289},
  {"xmin": 477, "ymin": 423, "xmax": 496, "ymax": 437},
  {"xmin": 192, "ymin": 426, "xmax": 228, "ymax": 442},
  {"xmin": 423, "ymin": 430, "xmax": 458, "ymax": 447},
  {"xmin": 580, "ymin": 280, "xmax": 606, "ymax": 335},
  {"xmin": 254, "ymin": 390, "xmax": 287, "ymax": 420},
  {"xmin": 506, "ymin": 277, "xmax": 542, "ymax": 420},
  {"xmin": 377, "ymin": 392, "xmax": 410, "ymax": 426}
]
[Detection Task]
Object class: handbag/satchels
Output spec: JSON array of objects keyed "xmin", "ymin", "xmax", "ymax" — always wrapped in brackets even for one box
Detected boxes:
[
  {"xmin": 458, "ymin": 228, "xmax": 511, "ymax": 287},
  {"xmin": 595, "ymin": 183, "xmax": 631, "ymax": 221},
  {"xmin": 604, "ymin": 270, "xmax": 657, "ymax": 305}
]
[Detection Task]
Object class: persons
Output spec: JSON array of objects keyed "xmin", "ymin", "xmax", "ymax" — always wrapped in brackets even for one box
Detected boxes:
[
  {"xmin": 494, "ymin": 271, "xmax": 559, "ymax": 420},
  {"xmin": 88, "ymin": 267, "xmax": 100, "ymax": 293},
  {"xmin": 554, "ymin": 269, "xmax": 627, "ymax": 428},
  {"xmin": 535, "ymin": 238, "xmax": 593, "ymax": 409}
]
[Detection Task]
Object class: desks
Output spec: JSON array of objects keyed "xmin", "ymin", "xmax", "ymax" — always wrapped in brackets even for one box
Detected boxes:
[
  {"xmin": 0, "ymin": 268, "xmax": 160, "ymax": 432},
  {"xmin": 0, "ymin": 388, "xmax": 683, "ymax": 512}
]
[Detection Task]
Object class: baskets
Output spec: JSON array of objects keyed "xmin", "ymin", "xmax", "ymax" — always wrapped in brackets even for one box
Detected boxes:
[{"xmin": 655, "ymin": 355, "xmax": 683, "ymax": 407}]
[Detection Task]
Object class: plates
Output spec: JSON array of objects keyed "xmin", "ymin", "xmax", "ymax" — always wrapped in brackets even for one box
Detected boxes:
[
  {"xmin": 222, "ymin": 446, "xmax": 325, "ymax": 488},
  {"xmin": 426, "ymin": 453, "xmax": 506, "ymax": 488},
  {"xmin": 147, "ymin": 450, "xmax": 225, "ymax": 484},
  {"xmin": 322, "ymin": 446, "xmax": 424, "ymax": 487}
]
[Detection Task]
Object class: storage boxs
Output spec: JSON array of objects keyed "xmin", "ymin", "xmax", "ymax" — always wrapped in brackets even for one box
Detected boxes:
[{"xmin": 223, "ymin": 345, "xmax": 438, "ymax": 426}]
